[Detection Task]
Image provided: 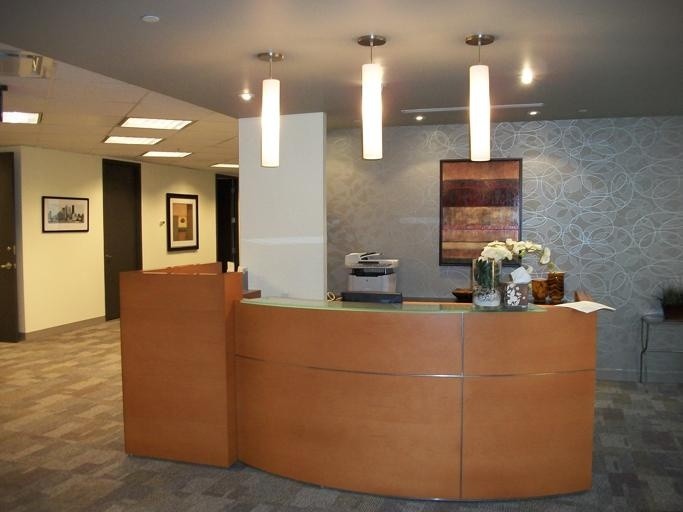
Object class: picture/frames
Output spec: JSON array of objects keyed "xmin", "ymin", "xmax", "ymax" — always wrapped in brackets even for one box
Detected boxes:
[
  {"xmin": 438, "ymin": 158, "xmax": 522, "ymax": 266},
  {"xmin": 41, "ymin": 196, "xmax": 89, "ymax": 233},
  {"xmin": 165, "ymin": 193, "xmax": 200, "ymax": 253}
]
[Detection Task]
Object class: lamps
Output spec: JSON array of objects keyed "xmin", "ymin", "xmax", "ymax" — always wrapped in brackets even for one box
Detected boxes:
[
  {"xmin": 463, "ymin": 33, "xmax": 496, "ymax": 162},
  {"xmin": 256, "ymin": 52, "xmax": 286, "ymax": 170},
  {"xmin": 356, "ymin": 34, "xmax": 386, "ymax": 159}
]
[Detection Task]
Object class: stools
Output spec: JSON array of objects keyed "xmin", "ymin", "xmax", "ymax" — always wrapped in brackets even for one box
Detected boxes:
[{"xmin": 638, "ymin": 314, "xmax": 683, "ymax": 385}]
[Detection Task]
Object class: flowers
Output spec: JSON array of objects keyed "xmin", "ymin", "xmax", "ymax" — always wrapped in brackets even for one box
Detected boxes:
[{"xmin": 472, "ymin": 237, "xmax": 550, "ymax": 287}]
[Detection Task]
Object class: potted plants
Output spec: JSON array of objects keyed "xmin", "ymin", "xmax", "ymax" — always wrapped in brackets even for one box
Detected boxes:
[{"xmin": 652, "ymin": 279, "xmax": 683, "ymax": 322}]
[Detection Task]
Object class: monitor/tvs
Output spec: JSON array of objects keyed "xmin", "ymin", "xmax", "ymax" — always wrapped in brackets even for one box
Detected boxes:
[{"xmin": 340, "ymin": 291, "xmax": 402, "ymax": 304}]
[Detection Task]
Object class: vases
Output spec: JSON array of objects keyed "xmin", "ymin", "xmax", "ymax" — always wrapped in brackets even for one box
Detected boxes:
[
  {"xmin": 471, "ymin": 285, "xmax": 501, "ymax": 307},
  {"xmin": 499, "ymin": 282, "xmax": 528, "ymax": 311}
]
